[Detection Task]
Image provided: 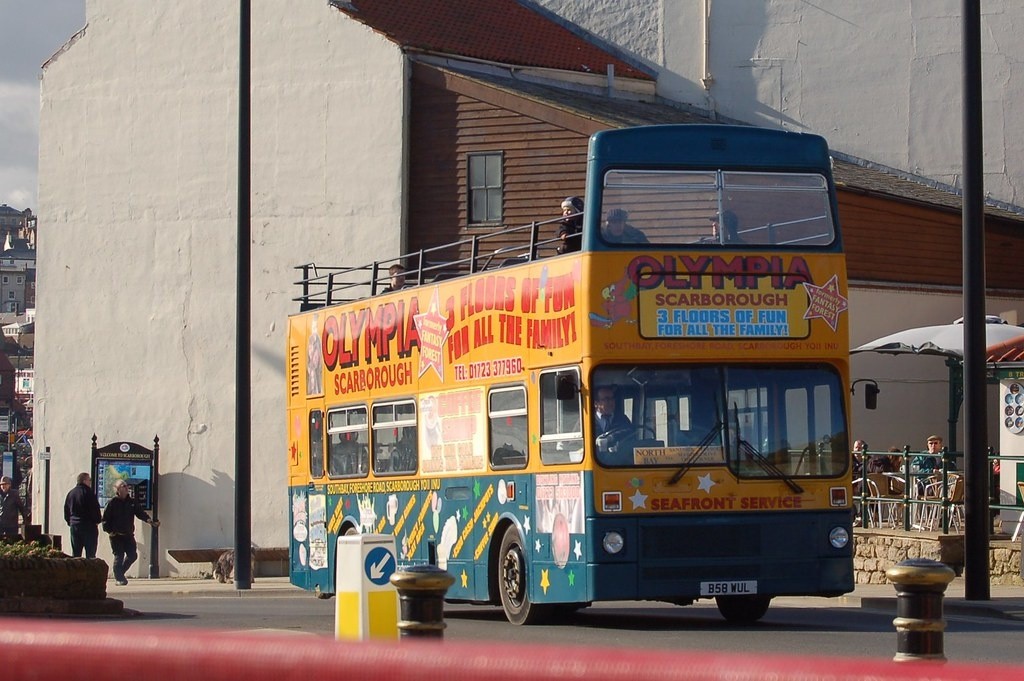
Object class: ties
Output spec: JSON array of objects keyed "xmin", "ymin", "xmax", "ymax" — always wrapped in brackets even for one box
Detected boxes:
[{"xmin": 602, "ymin": 415, "xmax": 611, "ymax": 433}]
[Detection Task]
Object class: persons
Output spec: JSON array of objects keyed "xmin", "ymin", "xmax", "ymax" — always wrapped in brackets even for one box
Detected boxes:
[
  {"xmin": 601, "ymin": 209, "xmax": 650, "ymax": 244},
  {"xmin": 851, "ymin": 441, "xmax": 877, "ymax": 525},
  {"xmin": 381, "ymin": 264, "xmax": 406, "ymax": 293},
  {"xmin": 912, "ymin": 436, "xmax": 959, "ymax": 495},
  {"xmin": 553, "ymin": 197, "xmax": 584, "ymax": 255},
  {"xmin": 0, "ymin": 476, "xmax": 30, "ymax": 540},
  {"xmin": 63, "ymin": 473, "xmax": 101, "ymax": 558},
  {"xmin": 857, "ymin": 449, "xmax": 903, "ymax": 492},
  {"xmin": 568, "ymin": 389, "xmax": 634, "ymax": 450},
  {"xmin": 700, "ymin": 211, "xmax": 744, "ymax": 244},
  {"xmin": 101, "ymin": 480, "xmax": 161, "ymax": 584}
]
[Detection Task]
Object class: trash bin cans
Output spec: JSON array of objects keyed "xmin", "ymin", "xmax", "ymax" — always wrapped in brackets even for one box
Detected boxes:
[{"xmin": 334, "ymin": 532, "xmax": 401, "ymax": 643}]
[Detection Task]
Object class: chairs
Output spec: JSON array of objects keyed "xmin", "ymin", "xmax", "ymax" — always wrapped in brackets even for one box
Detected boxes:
[
  {"xmin": 1011, "ymin": 482, "xmax": 1024, "ymax": 543},
  {"xmin": 331, "ymin": 427, "xmax": 418, "ymax": 473},
  {"xmin": 851, "ymin": 471, "xmax": 965, "ymax": 534}
]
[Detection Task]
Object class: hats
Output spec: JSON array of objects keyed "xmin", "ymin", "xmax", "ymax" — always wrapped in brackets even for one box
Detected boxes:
[
  {"xmin": 0, "ymin": 476, "xmax": 12, "ymax": 485},
  {"xmin": 560, "ymin": 197, "xmax": 584, "ymax": 213},
  {"xmin": 605, "ymin": 208, "xmax": 628, "ymax": 221},
  {"xmin": 709, "ymin": 210, "xmax": 738, "ymax": 229}
]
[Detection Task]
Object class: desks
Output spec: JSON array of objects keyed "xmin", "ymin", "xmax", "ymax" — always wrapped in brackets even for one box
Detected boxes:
[{"xmin": 881, "ymin": 473, "xmax": 938, "ymax": 532}]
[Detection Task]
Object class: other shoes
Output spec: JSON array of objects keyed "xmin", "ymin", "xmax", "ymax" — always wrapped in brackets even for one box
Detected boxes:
[{"xmin": 116, "ymin": 578, "xmax": 128, "ymax": 586}]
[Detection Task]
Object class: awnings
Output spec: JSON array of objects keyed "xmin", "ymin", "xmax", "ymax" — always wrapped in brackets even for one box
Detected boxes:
[{"xmin": 849, "ymin": 324, "xmax": 1024, "ymax": 365}]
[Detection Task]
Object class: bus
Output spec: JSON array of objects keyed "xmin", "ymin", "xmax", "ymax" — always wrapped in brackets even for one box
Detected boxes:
[{"xmin": 285, "ymin": 126, "xmax": 880, "ymax": 627}]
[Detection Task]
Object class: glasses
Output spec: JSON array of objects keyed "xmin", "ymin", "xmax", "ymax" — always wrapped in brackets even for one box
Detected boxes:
[
  {"xmin": 120, "ymin": 484, "xmax": 129, "ymax": 489},
  {"xmin": 599, "ymin": 396, "xmax": 615, "ymax": 403}
]
